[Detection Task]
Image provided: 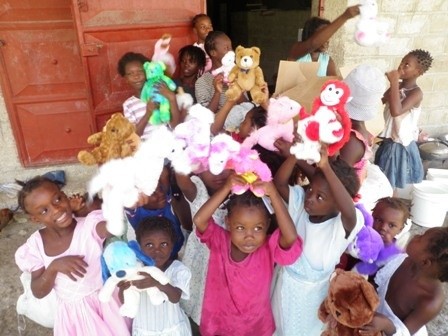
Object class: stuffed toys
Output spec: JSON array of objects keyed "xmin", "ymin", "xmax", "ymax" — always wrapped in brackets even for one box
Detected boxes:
[
  {"xmin": 188, "ymin": 125, "xmax": 272, "ymax": 198},
  {"xmin": 289, "ymin": 78, "xmax": 353, "ymax": 165},
  {"xmin": 139, "ymin": 60, "xmax": 177, "ymax": 127},
  {"xmin": 318, "ymin": 267, "xmax": 382, "ymax": 336},
  {"xmin": 150, "ymin": 33, "xmax": 176, "ymax": 76},
  {"xmin": 174, "ymin": 103, "xmax": 215, "ymax": 145},
  {"xmin": 97, "ymin": 239, "xmax": 173, "ymax": 320},
  {"xmin": 241, "ymin": 95, "xmax": 301, "ymax": 153},
  {"xmin": 212, "ymin": 50, "xmax": 237, "ymax": 85},
  {"xmin": 224, "ymin": 44, "xmax": 268, "ymax": 105},
  {"xmin": 345, "ymin": 202, "xmax": 405, "ymax": 276},
  {"xmin": 85, "ymin": 123, "xmax": 186, "ymax": 237},
  {"xmin": 76, "ymin": 111, "xmax": 141, "ymax": 165}
]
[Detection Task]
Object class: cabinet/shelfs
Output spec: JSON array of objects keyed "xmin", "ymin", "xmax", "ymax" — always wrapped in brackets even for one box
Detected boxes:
[{"xmin": 0, "ymin": 0, "xmax": 207, "ymax": 169}]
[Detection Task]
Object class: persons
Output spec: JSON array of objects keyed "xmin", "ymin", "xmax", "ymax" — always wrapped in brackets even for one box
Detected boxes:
[
  {"xmin": 13, "ymin": 172, "xmax": 149, "ymax": 335},
  {"xmin": 210, "ymin": 91, "xmax": 268, "ymax": 144},
  {"xmin": 191, "ymin": 14, "xmax": 214, "ymax": 72},
  {"xmin": 173, "ymin": 153, "xmax": 236, "ymax": 327},
  {"xmin": 337, "ymin": 62, "xmax": 392, "ymax": 184},
  {"xmin": 116, "ymin": 214, "xmax": 192, "ymax": 336},
  {"xmin": 123, "ymin": 160, "xmax": 193, "ymax": 264},
  {"xmin": 68, "ymin": 189, "xmax": 104, "ymax": 217},
  {"xmin": 172, "ymin": 45, "xmax": 207, "ymax": 103},
  {"xmin": 191, "ymin": 170, "xmax": 305, "ymax": 336},
  {"xmin": 268, "ymin": 121, "xmax": 365, "ymax": 336},
  {"xmin": 358, "ymin": 225, "xmax": 448, "ymax": 336},
  {"xmin": 286, "ymin": 2, "xmax": 364, "ymax": 77},
  {"xmin": 194, "ymin": 30, "xmax": 250, "ymax": 113},
  {"xmin": 374, "ymin": 47, "xmax": 434, "ymax": 188},
  {"xmin": 113, "ymin": 50, "xmax": 188, "ymax": 139},
  {"xmin": 336, "ymin": 196, "xmax": 415, "ymax": 289}
]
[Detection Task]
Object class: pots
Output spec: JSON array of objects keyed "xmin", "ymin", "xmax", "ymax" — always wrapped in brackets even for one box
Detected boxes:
[{"xmin": 418, "ymin": 140, "xmax": 448, "ymax": 162}]
